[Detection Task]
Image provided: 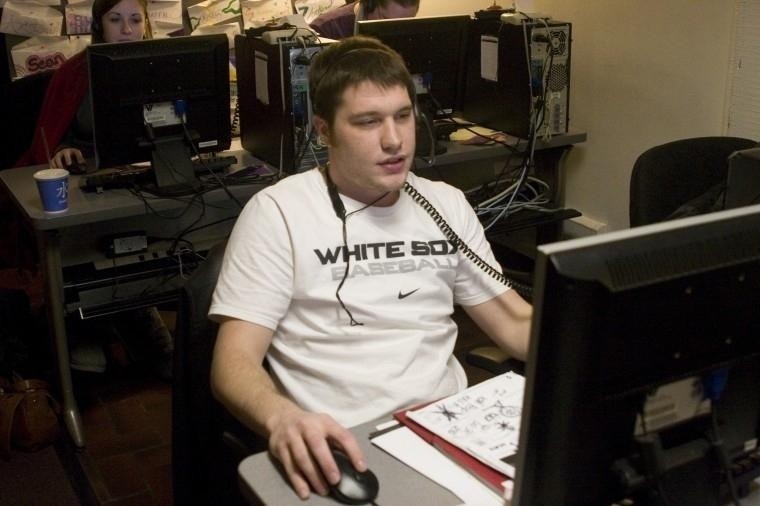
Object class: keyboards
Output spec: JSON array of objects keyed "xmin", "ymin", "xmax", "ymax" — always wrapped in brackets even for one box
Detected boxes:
[
  {"xmin": 84, "ymin": 156, "xmax": 238, "ymax": 195},
  {"xmin": 434, "ymin": 107, "xmax": 454, "ymax": 118}
]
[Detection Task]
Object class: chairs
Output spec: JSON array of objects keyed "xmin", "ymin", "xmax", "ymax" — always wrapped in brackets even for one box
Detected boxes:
[{"xmin": 630, "ymin": 136, "xmax": 754, "ymax": 234}]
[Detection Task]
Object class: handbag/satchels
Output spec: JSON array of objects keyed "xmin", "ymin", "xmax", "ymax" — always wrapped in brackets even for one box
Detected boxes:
[{"xmin": 0, "ymin": 370, "xmax": 62, "ymax": 463}]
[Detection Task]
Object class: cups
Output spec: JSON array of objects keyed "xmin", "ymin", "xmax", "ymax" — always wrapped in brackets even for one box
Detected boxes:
[{"xmin": 32, "ymin": 167, "xmax": 71, "ymax": 215}]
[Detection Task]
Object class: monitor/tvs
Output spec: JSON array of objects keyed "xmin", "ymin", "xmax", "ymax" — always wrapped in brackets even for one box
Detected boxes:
[
  {"xmin": 85, "ymin": 32, "xmax": 233, "ymax": 195},
  {"xmin": 357, "ymin": 15, "xmax": 482, "ymax": 154},
  {"xmin": 516, "ymin": 202, "xmax": 760, "ymax": 506}
]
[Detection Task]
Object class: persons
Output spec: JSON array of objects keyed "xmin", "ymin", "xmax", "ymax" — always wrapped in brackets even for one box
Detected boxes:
[
  {"xmin": 206, "ymin": 34, "xmax": 535, "ymax": 501},
  {"xmin": 30, "ymin": 0, "xmax": 174, "ymax": 373},
  {"xmin": 308, "ymin": 0, "xmax": 420, "ymax": 42}
]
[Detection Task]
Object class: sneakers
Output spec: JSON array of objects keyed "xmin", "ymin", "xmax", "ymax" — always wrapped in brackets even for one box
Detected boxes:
[{"xmin": 69, "ymin": 336, "xmax": 108, "ymax": 374}]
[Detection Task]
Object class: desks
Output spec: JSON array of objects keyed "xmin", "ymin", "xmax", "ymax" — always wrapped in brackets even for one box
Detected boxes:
[
  {"xmin": 1, "ymin": 136, "xmax": 290, "ymax": 447},
  {"xmin": 237, "ymin": 412, "xmax": 466, "ymax": 506},
  {"xmin": 411, "ymin": 126, "xmax": 587, "ymax": 245}
]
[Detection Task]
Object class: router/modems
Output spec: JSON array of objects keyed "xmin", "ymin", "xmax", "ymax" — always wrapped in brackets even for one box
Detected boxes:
[
  {"xmin": 262, "ymin": 29, "xmax": 320, "ymax": 47},
  {"xmin": 499, "ymin": 10, "xmax": 551, "ymax": 26}
]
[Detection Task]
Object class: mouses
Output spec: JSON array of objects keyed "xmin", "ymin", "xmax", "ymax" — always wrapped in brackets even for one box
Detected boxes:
[
  {"xmin": 318, "ymin": 442, "xmax": 380, "ymax": 506},
  {"xmin": 67, "ymin": 161, "xmax": 91, "ymax": 175}
]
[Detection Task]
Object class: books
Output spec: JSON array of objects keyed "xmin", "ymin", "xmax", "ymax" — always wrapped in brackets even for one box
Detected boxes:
[{"xmin": 393, "ymin": 383, "xmax": 515, "ymax": 502}]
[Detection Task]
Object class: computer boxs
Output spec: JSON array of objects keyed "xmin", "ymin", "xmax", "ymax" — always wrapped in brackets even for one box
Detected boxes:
[
  {"xmin": 461, "ymin": 16, "xmax": 572, "ymax": 137},
  {"xmin": 234, "ymin": 33, "xmax": 340, "ymax": 176}
]
